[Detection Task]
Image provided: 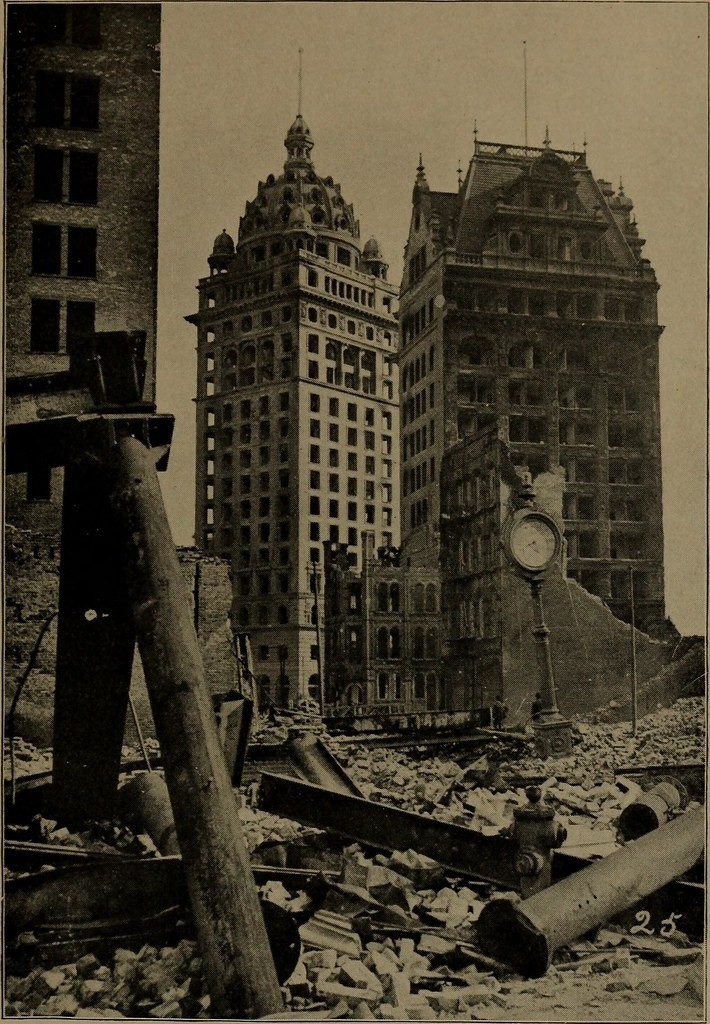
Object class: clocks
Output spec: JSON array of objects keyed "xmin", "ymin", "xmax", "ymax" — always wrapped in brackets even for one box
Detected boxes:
[{"xmin": 507, "ymin": 508, "xmax": 565, "ymax": 568}]
